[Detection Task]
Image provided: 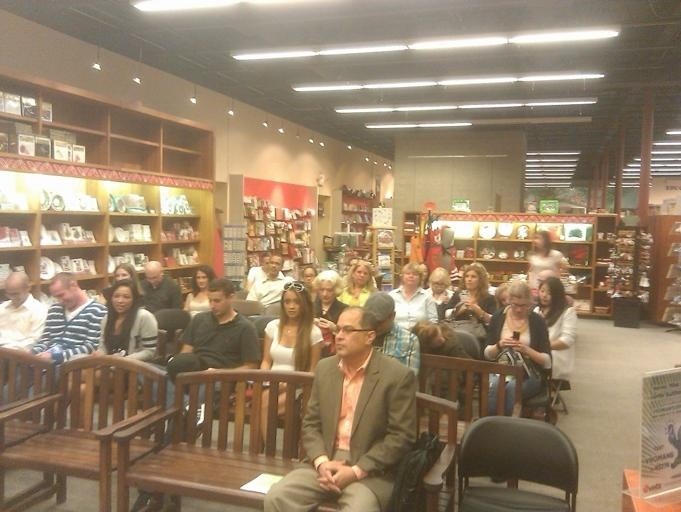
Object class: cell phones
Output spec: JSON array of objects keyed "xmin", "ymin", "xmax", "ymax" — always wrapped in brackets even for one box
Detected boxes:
[{"xmin": 513, "ymin": 330, "xmax": 520, "ymax": 340}]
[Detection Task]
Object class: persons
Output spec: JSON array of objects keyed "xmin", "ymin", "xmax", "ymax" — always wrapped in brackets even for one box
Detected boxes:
[
  {"xmin": 481, "ymin": 280, "xmax": 552, "ymax": 416},
  {"xmin": 532, "ymin": 271, "xmax": 573, "ymax": 310},
  {"xmin": 443, "ymin": 266, "xmax": 497, "ymax": 327},
  {"xmin": 54, "ymin": 260, "xmax": 183, "ymax": 428},
  {"xmin": 303, "ymin": 260, "xmax": 379, "ymax": 356},
  {"xmin": 533, "ymin": 277, "xmax": 577, "ymax": 424},
  {"xmin": 259, "ymin": 282, "xmax": 324, "ymax": 454},
  {"xmin": 247, "ymin": 254, "xmax": 294, "ymax": 336},
  {"xmin": 163, "ymin": 263, "xmax": 262, "ymax": 440},
  {"xmin": 364, "ymin": 262, "xmax": 452, "ymax": 369},
  {"xmin": 264, "ymin": 306, "xmax": 417, "ymax": 512},
  {"xmin": 414, "ymin": 321, "xmax": 480, "ymax": 418},
  {"xmin": 0, "ymin": 272, "xmax": 109, "ymax": 403},
  {"xmin": 527, "ymin": 232, "xmax": 570, "ymax": 297}
]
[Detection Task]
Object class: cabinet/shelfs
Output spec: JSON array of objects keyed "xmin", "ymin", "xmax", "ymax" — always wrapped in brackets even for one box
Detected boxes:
[
  {"xmin": 330, "ymin": 189, "xmax": 375, "ymax": 251},
  {"xmin": 367, "ymin": 226, "xmax": 397, "ymax": 290},
  {"xmin": 0, "ymin": 64, "xmax": 214, "ymax": 299},
  {"xmin": 425, "ymin": 212, "xmax": 620, "ymax": 320}
]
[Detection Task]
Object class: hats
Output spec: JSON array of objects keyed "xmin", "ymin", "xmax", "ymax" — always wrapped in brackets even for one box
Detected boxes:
[{"xmin": 361, "ymin": 290, "xmax": 396, "ymax": 322}]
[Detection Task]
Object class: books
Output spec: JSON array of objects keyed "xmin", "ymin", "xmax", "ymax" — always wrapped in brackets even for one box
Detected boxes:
[{"xmin": 0, "ymin": 91, "xmax": 85, "ymax": 162}]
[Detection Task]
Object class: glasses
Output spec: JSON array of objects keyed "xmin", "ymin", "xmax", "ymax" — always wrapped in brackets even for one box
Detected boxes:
[
  {"xmin": 332, "ymin": 325, "xmax": 370, "ymax": 335},
  {"xmin": 283, "ymin": 282, "xmax": 305, "ymax": 294}
]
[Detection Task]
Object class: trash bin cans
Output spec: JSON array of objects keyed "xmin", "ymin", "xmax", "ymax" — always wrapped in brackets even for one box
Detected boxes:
[{"xmin": 611, "ymin": 297, "xmax": 641, "ymax": 329}]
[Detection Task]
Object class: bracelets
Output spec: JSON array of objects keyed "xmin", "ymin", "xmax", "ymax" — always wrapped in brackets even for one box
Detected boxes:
[{"xmin": 497, "ymin": 341, "xmax": 500, "ymax": 349}]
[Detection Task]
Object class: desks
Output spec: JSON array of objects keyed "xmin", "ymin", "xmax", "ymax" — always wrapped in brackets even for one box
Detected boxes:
[{"xmin": 619, "ymin": 464, "xmax": 680, "ymax": 512}]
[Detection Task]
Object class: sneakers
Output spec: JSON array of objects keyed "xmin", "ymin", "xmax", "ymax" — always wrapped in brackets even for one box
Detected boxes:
[{"xmin": 187, "ymin": 402, "xmax": 206, "ymax": 432}]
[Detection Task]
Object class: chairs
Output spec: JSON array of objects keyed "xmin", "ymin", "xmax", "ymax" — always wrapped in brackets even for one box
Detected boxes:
[{"xmin": 0, "ymin": 297, "xmax": 578, "ymax": 512}]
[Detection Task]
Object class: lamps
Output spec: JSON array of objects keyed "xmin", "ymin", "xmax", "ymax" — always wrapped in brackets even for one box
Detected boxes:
[
  {"xmin": 334, "ymin": 97, "xmax": 597, "ymax": 117},
  {"xmin": 230, "ymin": 26, "xmax": 620, "ymax": 60},
  {"xmin": 89, "ymin": 20, "xmax": 102, "ymax": 72},
  {"xmin": 290, "ymin": 70, "xmax": 608, "ymax": 92},
  {"xmin": 128, "ymin": 0, "xmax": 239, "ymax": 13},
  {"xmin": 363, "ymin": 116, "xmax": 594, "ymax": 128}
]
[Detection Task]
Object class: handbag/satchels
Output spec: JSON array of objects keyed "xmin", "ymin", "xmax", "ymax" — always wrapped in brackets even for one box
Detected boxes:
[
  {"xmin": 389, "ymin": 430, "xmax": 442, "ymax": 512},
  {"xmin": 492, "ymin": 347, "xmax": 534, "ymax": 380}
]
